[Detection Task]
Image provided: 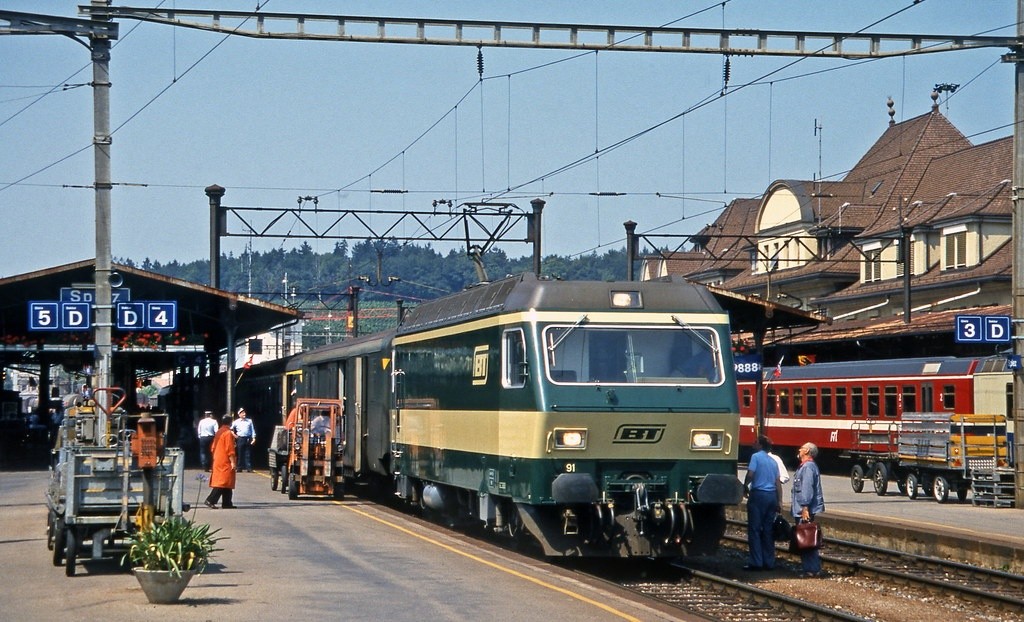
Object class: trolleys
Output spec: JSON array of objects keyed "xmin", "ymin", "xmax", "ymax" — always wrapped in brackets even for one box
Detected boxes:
[{"xmin": 42, "ymin": 383, "xmax": 191, "ymax": 578}]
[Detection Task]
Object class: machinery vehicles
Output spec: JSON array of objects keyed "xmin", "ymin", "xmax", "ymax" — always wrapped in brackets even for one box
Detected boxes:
[{"xmin": 268, "ymin": 397, "xmax": 349, "ymax": 501}]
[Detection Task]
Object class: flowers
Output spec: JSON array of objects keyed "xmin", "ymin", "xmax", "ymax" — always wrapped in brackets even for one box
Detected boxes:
[{"xmin": 118, "ymin": 472, "xmax": 231, "ymax": 580}]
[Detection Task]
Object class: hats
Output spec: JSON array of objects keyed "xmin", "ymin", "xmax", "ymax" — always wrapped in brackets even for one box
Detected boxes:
[
  {"xmin": 238, "ymin": 408, "xmax": 244, "ymax": 415},
  {"xmin": 205, "ymin": 411, "xmax": 212, "ymax": 414}
]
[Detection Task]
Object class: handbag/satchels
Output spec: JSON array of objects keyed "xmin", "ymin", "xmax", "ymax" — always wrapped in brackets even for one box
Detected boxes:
[
  {"xmin": 790, "ymin": 518, "xmax": 824, "ymax": 555},
  {"xmin": 774, "ymin": 512, "xmax": 788, "ymax": 535}
]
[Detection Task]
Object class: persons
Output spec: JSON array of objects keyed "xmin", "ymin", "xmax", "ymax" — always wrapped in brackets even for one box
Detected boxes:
[
  {"xmin": 197, "ymin": 411, "xmax": 219, "ymax": 471},
  {"xmin": 204, "ymin": 414, "xmax": 237, "ymax": 509},
  {"xmin": 286, "ymin": 404, "xmax": 306, "ymax": 428},
  {"xmin": 51, "ymin": 406, "xmax": 63, "ymax": 433},
  {"xmin": 767, "ymin": 446, "xmax": 790, "ymax": 484},
  {"xmin": 790, "ymin": 442, "xmax": 826, "ymax": 578},
  {"xmin": 230, "ymin": 408, "xmax": 256, "ymax": 473},
  {"xmin": 744, "ymin": 435, "xmax": 782, "ymax": 572},
  {"xmin": 312, "ymin": 411, "xmax": 330, "ymax": 433}
]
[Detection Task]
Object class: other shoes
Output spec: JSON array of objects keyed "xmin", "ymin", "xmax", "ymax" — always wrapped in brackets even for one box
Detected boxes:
[
  {"xmin": 799, "ymin": 570, "xmax": 822, "ymax": 578},
  {"xmin": 743, "ymin": 565, "xmax": 762, "ymax": 571},
  {"xmin": 204, "ymin": 500, "xmax": 218, "ymax": 509},
  {"xmin": 223, "ymin": 506, "xmax": 237, "ymax": 509},
  {"xmin": 764, "ymin": 566, "xmax": 777, "ymax": 570}
]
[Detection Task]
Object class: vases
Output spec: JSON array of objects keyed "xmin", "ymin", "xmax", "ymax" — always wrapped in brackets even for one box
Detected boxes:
[{"xmin": 130, "ymin": 565, "xmax": 197, "ymax": 604}]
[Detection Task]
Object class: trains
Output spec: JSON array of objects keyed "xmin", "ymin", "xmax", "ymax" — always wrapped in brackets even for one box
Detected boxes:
[
  {"xmin": 731, "ymin": 351, "xmax": 1013, "ymax": 465},
  {"xmin": 154, "ymin": 270, "xmax": 747, "ymax": 571}
]
